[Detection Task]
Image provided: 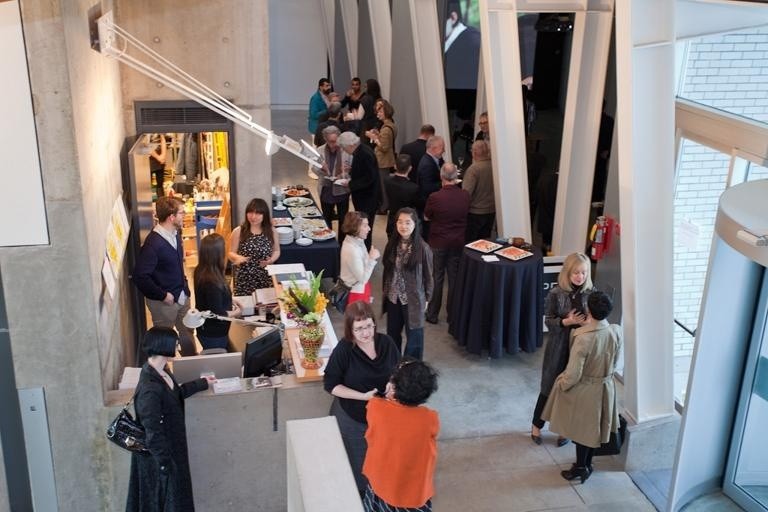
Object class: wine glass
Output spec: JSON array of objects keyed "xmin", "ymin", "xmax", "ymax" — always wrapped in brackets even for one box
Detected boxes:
[{"xmin": 457, "ymin": 154, "xmax": 464, "ymax": 168}]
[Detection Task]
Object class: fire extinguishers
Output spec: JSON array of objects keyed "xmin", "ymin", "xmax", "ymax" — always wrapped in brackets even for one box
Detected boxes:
[{"xmin": 590, "ymin": 216, "xmax": 607, "ymax": 260}]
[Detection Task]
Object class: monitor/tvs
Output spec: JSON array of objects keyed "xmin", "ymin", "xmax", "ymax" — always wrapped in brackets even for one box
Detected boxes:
[
  {"xmin": 243, "ymin": 321, "xmax": 285, "ymax": 378},
  {"xmin": 172, "ymin": 352, "xmax": 244, "ymax": 385}
]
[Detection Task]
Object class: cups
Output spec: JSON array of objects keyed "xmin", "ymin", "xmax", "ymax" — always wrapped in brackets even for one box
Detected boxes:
[
  {"xmin": 293, "ymin": 228, "xmax": 302, "ymax": 239},
  {"xmin": 199, "ymin": 228, "xmax": 215, "ymax": 238}
]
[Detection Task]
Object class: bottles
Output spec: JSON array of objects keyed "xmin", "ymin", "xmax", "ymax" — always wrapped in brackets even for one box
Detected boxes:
[
  {"xmin": 150, "ymin": 172, "xmax": 158, "ymax": 202},
  {"xmin": 493, "ymin": 236, "xmax": 528, "ymax": 247}
]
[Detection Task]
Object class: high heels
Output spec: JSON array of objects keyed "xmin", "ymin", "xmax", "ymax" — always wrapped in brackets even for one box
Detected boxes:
[{"xmin": 532, "ymin": 425, "xmax": 593, "ymax": 484}]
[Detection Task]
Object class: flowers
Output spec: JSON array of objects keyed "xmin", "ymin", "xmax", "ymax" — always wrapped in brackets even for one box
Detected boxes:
[{"xmin": 276, "ymin": 267, "xmax": 331, "ymax": 330}]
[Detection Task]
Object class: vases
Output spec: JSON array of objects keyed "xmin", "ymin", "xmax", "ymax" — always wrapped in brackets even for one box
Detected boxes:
[{"xmin": 298, "ymin": 323, "xmax": 325, "ymax": 369}]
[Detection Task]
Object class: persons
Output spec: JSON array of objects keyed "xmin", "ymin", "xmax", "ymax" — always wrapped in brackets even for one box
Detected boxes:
[
  {"xmin": 131, "ymin": 131, "xmax": 284, "ymax": 358},
  {"xmin": 308, "ymin": 77, "xmax": 398, "ymax": 254},
  {"xmin": 444, "ymin": 0, "xmax": 483, "ymax": 89},
  {"xmin": 527, "ymin": 252, "xmax": 598, "ymax": 449},
  {"xmin": 390, "ymin": 111, "xmax": 497, "ymax": 326},
  {"xmin": 320, "ymin": 301, "xmax": 402, "ymax": 497},
  {"xmin": 359, "ymin": 359, "xmax": 444, "ymax": 511},
  {"xmin": 331, "ymin": 210, "xmax": 379, "ymax": 329},
  {"xmin": 556, "ymin": 289, "xmax": 623, "ymax": 485},
  {"xmin": 382, "ymin": 206, "xmax": 436, "ymax": 364},
  {"xmin": 123, "ymin": 326, "xmax": 219, "ymax": 511}
]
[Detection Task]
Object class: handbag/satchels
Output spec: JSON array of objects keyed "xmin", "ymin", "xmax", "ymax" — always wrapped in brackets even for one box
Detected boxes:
[
  {"xmin": 328, "ymin": 279, "xmax": 352, "ymax": 315},
  {"xmin": 105, "ymin": 408, "xmax": 150, "ymax": 457},
  {"xmin": 593, "ymin": 414, "xmax": 626, "ymax": 456}
]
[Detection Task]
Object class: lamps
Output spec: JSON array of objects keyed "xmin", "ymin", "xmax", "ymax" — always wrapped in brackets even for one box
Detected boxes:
[
  {"xmin": 94, "ymin": 9, "xmax": 326, "ymax": 181},
  {"xmin": 181, "ymin": 306, "xmax": 292, "ymax": 377}
]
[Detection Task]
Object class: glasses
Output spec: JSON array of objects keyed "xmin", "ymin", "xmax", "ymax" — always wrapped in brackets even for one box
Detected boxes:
[{"xmin": 351, "ymin": 323, "xmax": 376, "ymax": 333}]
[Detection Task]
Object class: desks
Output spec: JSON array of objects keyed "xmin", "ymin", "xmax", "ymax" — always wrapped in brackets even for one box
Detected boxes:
[{"xmin": 446, "ymin": 240, "xmax": 546, "ymax": 358}]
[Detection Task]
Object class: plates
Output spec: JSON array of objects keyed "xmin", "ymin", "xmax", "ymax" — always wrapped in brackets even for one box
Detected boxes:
[
  {"xmin": 295, "ymin": 237, "xmax": 313, "ymax": 247},
  {"xmin": 302, "ymin": 228, "xmax": 336, "ymax": 242},
  {"xmin": 332, "ymin": 178, "xmax": 352, "ymax": 186},
  {"xmin": 272, "ymin": 187, "xmax": 328, "ymax": 229},
  {"xmin": 464, "ymin": 239, "xmax": 503, "ymax": 255},
  {"xmin": 275, "ymin": 227, "xmax": 294, "ymax": 245},
  {"xmin": 494, "ymin": 247, "xmax": 534, "ymax": 262}
]
